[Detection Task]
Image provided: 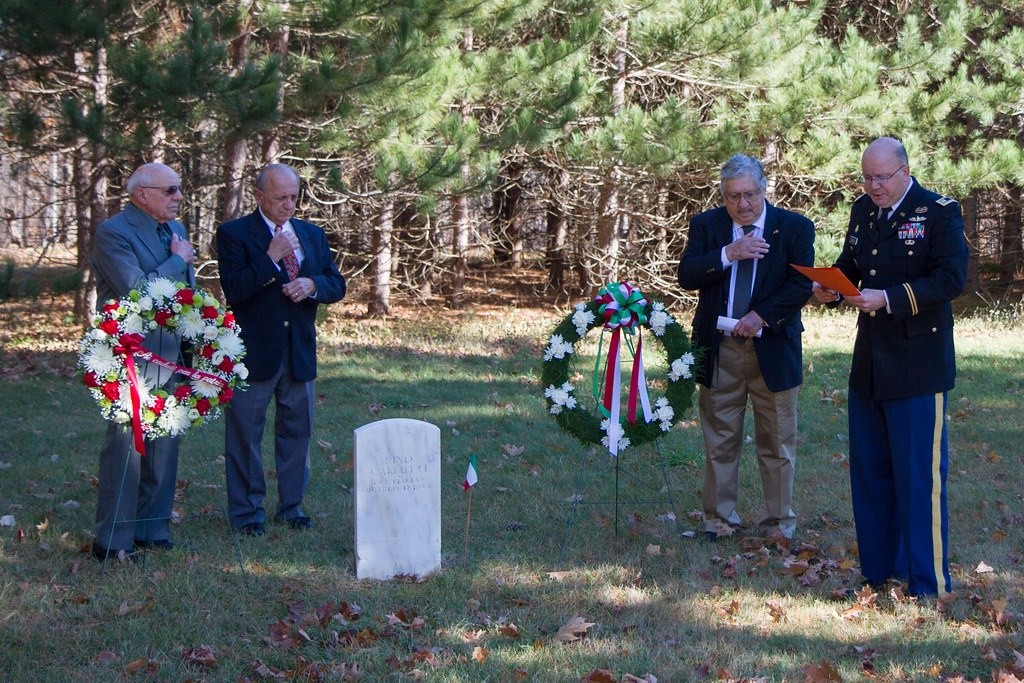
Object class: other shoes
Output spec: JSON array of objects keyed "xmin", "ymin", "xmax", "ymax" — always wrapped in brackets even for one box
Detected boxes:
[
  {"xmin": 275, "ymin": 515, "xmax": 312, "ymax": 531},
  {"xmin": 134, "ymin": 538, "xmax": 190, "ymax": 550},
  {"xmin": 242, "ymin": 521, "xmax": 265, "ymax": 537},
  {"xmin": 836, "ymin": 579, "xmax": 884, "ymax": 601},
  {"xmin": 92, "ymin": 541, "xmax": 142, "ymax": 564},
  {"xmin": 702, "ymin": 523, "xmax": 742, "ymax": 542}
]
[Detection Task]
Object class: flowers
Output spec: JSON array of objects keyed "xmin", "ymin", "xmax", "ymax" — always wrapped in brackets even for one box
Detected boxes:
[
  {"xmin": 75, "ymin": 277, "xmax": 250, "ymax": 443},
  {"xmin": 544, "ymin": 283, "xmax": 696, "ymax": 455}
]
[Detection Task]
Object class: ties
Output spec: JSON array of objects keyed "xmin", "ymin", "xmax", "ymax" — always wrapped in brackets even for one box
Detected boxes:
[
  {"xmin": 275, "ymin": 225, "xmax": 299, "ymax": 282},
  {"xmin": 876, "ymin": 207, "xmax": 893, "ymax": 233},
  {"xmin": 731, "ymin": 225, "xmax": 756, "ymax": 344},
  {"xmin": 156, "ymin": 223, "xmax": 173, "ymax": 259}
]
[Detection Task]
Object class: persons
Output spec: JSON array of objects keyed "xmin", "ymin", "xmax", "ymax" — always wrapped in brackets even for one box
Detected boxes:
[
  {"xmin": 90, "ymin": 163, "xmax": 201, "ymax": 564},
  {"xmin": 678, "ymin": 153, "xmax": 817, "ymax": 547},
  {"xmin": 812, "ymin": 136, "xmax": 970, "ymax": 614},
  {"xmin": 212, "ymin": 163, "xmax": 347, "ymax": 541}
]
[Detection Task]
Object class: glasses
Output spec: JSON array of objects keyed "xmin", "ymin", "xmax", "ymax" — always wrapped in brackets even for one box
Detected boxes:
[
  {"xmin": 139, "ymin": 185, "xmax": 183, "ymax": 195},
  {"xmin": 859, "ymin": 165, "xmax": 906, "ymax": 184},
  {"xmin": 722, "ymin": 187, "xmax": 761, "ymax": 204}
]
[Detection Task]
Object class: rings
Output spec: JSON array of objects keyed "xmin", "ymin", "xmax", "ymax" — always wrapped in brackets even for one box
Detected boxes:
[{"xmin": 295, "ymin": 293, "xmax": 299, "ymax": 296}]
